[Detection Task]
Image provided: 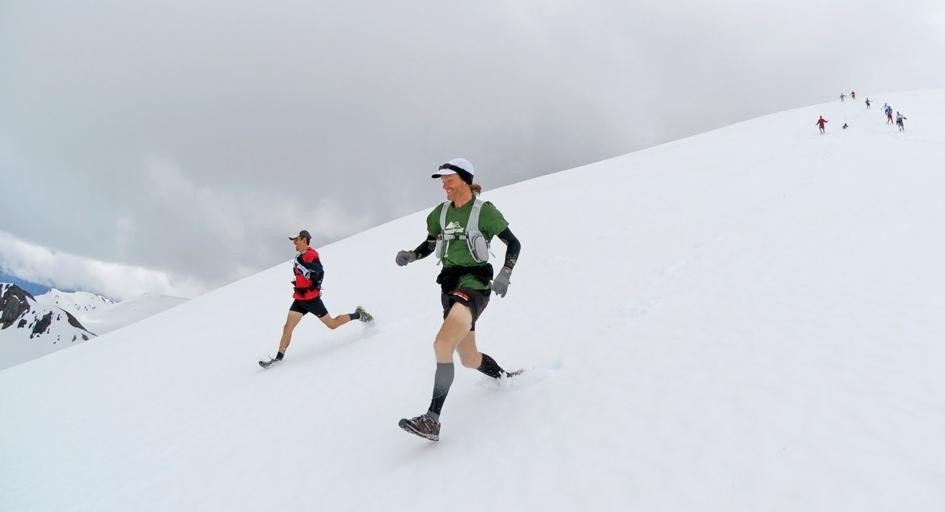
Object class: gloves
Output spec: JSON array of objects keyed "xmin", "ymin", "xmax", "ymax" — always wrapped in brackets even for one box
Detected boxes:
[
  {"xmin": 396, "ymin": 250, "xmax": 417, "ymax": 266},
  {"xmin": 492, "ymin": 270, "xmax": 511, "ymax": 297}
]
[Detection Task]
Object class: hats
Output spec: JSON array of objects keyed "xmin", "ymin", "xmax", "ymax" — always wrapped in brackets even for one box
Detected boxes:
[
  {"xmin": 289, "ymin": 228, "xmax": 311, "ymax": 240},
  {"xmin": 431, "ymin": 158, "xmax": 474, "ymax": 178}
]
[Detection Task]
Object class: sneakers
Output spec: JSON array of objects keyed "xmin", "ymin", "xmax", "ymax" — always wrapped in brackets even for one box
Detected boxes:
[
  {"xmin": 399, "ymin": 414, "xmax": 441, "ymax": 441},
  {"xmin": 259, "ymin": 359, "xmax": 280, "ymax": 368},
  {"xmin": 356, "ymin": 306, "xmax": 374, "ymax": 322}
]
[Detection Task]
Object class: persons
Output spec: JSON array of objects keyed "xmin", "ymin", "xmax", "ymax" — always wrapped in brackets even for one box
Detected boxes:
[
  {"xmin": 815, "ymin": 90, "xmax": 908, "ymax": 133},
  {"xmin": 396, "ymin": 158, "xmax": 524, "ymax": 441},
  {"xmin": 258, "ymin": 230, "xmax": 374, "ymax": 369}
]
